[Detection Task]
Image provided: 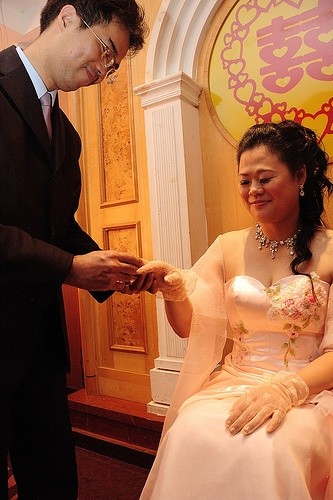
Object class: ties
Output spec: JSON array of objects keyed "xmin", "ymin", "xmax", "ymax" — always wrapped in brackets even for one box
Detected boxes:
[{"xmin": 40, "ymin": 92, "xmax": 52, "ymax": 143}]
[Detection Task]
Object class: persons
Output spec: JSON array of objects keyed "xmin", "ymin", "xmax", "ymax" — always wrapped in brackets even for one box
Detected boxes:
[
  {"xmin": 0, "ymin": 0, "xmax": 145, "ymax": 500},
  {"xmin": 137, "ymin": 121, "xmax": 333, "ymax": 500}
]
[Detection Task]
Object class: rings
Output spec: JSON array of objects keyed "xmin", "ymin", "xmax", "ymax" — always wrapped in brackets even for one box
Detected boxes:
[{"xmin": 129, "ymin": 276, "xmax": 135, "ymax": 287}]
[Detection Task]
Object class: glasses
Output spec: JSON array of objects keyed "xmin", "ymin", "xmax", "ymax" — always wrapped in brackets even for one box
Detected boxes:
[{"xmin": 62, "ymin": 11, "xmax": 117, "ymax": 84}]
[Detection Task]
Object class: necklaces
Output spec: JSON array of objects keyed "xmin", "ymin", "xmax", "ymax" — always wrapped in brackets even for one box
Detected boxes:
[{"xmin": 255, "ymin": 221, "xmax": 301, "ymax": 258}]
